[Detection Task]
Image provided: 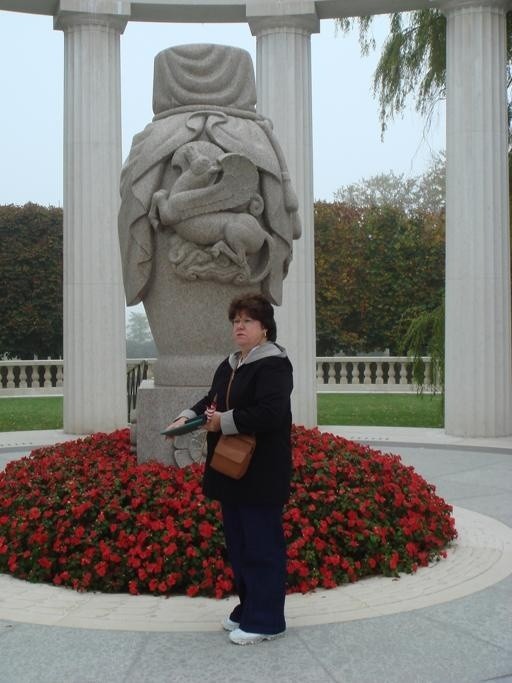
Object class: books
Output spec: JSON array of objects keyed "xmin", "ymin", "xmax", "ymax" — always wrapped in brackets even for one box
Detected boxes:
[{"xmin": 160, "ymin": 418, "xmax": 204, "ymax": 436}]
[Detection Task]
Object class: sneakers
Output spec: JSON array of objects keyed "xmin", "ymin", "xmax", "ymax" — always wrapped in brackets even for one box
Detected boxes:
[{"xmin": 221, "ymin": 616, "xmax": 286, "ymax": 646}]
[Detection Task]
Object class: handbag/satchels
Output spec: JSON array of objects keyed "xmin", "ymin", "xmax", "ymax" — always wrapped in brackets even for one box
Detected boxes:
[{"xmin": 210, "ymin": 431, "xmax": 257, "ymax": 480}]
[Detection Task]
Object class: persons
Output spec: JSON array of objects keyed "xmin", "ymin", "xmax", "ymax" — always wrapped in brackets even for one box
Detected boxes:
[{"xmin": 165, "ymin": 294, "xmax": 293, "ymax": 645}]
